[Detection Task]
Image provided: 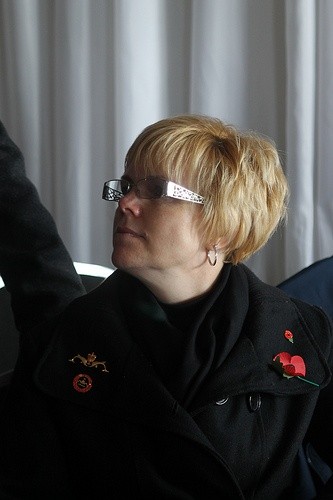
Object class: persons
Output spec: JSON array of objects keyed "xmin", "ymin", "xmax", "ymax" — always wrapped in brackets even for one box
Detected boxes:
[
  {"xmin": 0, "ymin": 114, "xmax": 333, "ymax": 500},
  {"xmin": 1, "ymin": 118, "xmax": 89, "ymax": 333}
]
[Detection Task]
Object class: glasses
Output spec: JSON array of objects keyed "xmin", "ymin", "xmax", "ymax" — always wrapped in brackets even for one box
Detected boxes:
[{"xmin": 102, "ymin": 176, "xmax": 204, "ymax": 204}]
